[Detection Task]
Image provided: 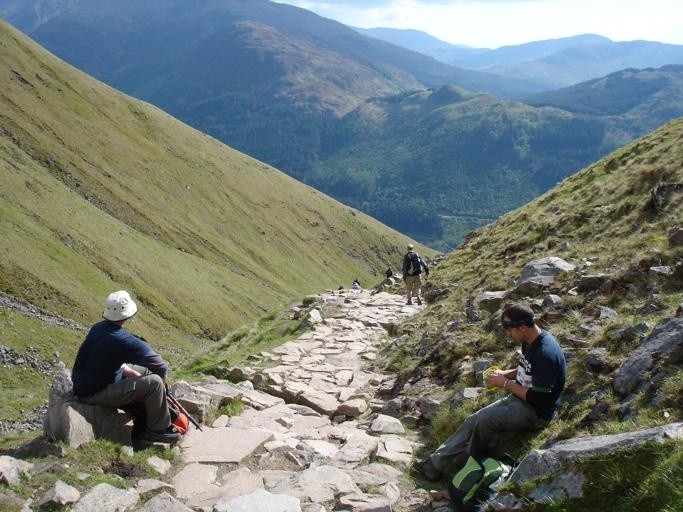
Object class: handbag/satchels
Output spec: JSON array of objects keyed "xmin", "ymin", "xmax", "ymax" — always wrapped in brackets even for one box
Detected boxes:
[
  {"xmin": 125, "ymin": 397, "xmax": 189, "ymax": 434},
  {"xmin": 448, "ymin": 453, "xmax": 521, "ymax": 512}
]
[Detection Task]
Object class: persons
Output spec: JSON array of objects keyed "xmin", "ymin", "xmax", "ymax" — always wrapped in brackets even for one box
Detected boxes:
[
  {"xmin": 385, "ymin": 267, "xmax": 392, "ymax": 278},
  {"xmin": 352, "ymin": 282, "xmax": 360, "ymax": 289},
  {"xmin": 352, "ymin": 278, "xmax": 360, "ymax": 286},
  {"xmin": 68, "ymin": 289, "xmax": 182, "ymax": 446},
  {"xmin": 410, "ymin": 300, "xmax": 567, "ymax": 486},
  {"xmin": 401, "ymin": 242, "xmax": 429, "ymax": 306}
]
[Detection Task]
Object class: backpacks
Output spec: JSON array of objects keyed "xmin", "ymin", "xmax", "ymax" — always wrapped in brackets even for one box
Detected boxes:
[{"xmin": 407, "ymin": 253, "xmax": 421, "ymax": 276}]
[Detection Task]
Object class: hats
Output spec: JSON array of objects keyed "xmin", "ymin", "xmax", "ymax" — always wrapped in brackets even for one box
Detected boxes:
[{"xmin": 103, "ymin": 290, "xmax": 138, "ymax": 322}]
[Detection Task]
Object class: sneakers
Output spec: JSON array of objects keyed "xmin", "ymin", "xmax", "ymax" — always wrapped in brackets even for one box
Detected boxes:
[
  {"xmin": 405, "ymin": 301, "xmax": 412, "ymax": 305},
  {"xmin": 413, "ymin": 458, "xmax": 442, "ymax": 482},
  {"xmin": 418, "ymin": 299, "xmax": 422, "ymax": 305},
  {"xmin": 143, "ymin": 427, "xmax": 182, "ymax": 442}
]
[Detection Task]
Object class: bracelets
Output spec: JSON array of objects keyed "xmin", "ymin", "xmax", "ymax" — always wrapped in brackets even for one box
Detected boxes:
[{"xmin": 503, "ymin": 377, "xmax": 508, "ymax": 391}]
